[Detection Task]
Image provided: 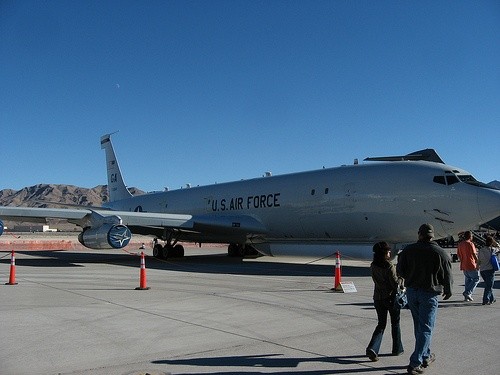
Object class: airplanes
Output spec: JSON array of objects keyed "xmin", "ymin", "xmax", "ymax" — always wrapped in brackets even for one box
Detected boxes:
[{"xmin": 0, "ymin": 130, "xmax": 500, "ymax": 260}]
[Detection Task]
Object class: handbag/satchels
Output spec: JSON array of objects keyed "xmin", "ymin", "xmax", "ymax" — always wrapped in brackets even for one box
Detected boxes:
[
  {"xmin": 390, "ymin": 277, "xmax": 409, "ymax": 309},
  {"xmin": 490, "ymin": 247, "xmax": 500, "ymax": 271}
]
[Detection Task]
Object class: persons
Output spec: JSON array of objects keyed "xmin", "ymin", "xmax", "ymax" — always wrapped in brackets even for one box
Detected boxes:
[
  {"xmin": 396, "ymin": 223, "xmax": 453, "ymax": 375},
  {"xmin": 366, "ymin": 242, "xmax": 406, "ymax": 362},
  {"xmin": 456, "ymin": 230, "xmax": 480, "ymax": 302},
  {"xmin": 477, "ymin": 236, "xmax": 500, "ymax": 305}
]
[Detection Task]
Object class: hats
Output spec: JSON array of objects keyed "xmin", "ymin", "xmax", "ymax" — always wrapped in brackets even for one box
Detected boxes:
[
  {"xmin": 372, "ymin": 241, "xmax": 392, "ymax": 252},
  {"xmin": 419, "ymin": 224, "xmax": 435, "ymax": 239}
]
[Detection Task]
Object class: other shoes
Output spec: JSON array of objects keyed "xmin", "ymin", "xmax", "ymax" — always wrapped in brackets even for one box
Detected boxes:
[
  {"xmin": 490, "ymin": 299, "xmax": 496, "ymax": 304},
  {"xmin": 407, "ymin": 367, "xmax": 424, "ymax": 375},
  {"xmin": 482, "ymin": 301, "xmax": 491, "ymax": 305},
  {"xmin": 392, "ymin": 350, "xmax": 405, "ymax": 356},
  {"xmin": 423, "ymin": 353, "xmax": 437, "ymax": 368},
  {"xmin": 366, "ymin": 347, "xmax": 379, "ymax": 362}
]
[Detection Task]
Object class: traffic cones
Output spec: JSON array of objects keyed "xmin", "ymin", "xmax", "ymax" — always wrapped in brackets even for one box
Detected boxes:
[
  {"xmin": 5, "ymin": 249, "xmax": 19, "ymax": 286},
  {"xmin": 332, "ymin": 252, "xmax": 344, "ymax": 291},
  {"xmin": 135, "ymin": 252, "xmax": 151, "ymax": 291}
]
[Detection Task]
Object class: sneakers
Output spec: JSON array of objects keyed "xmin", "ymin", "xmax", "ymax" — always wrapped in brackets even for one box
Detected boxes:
[
  {"xmin": 464, "ymin": 297, "xmax": 470, "ymax": 302},
  {"xmin": 462, "ymin": 291, "xmax": 474, "ymax": 301}
]
